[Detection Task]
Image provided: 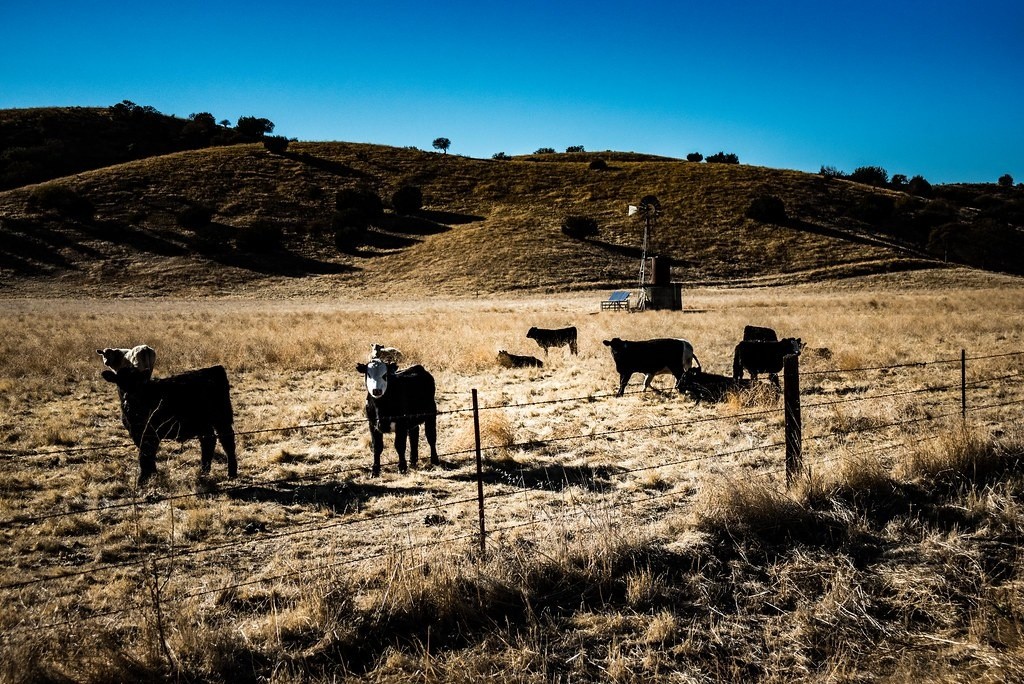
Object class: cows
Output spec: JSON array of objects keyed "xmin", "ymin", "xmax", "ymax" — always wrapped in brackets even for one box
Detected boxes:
[
  {"xmin": 366, "ymin": 342, "xmax": 408, "ymax": 364},
  {"xmin": 672, "ymin": 365, "xmax": 754, "ymax": 407},
  {"xmin": 601, "ymin": 336, "xmax": 702, "ymax": 399},
  {"xmin": 97, "ymin": 344, "xmax": 241, "ymax": 489},
  {"xmin": 740, "ymin": 324, "xmax": 780, "ymax": 343},
  {"xmin": 493, "ymin": 350, "xmax": 545, "ymax": 370},
  {"xmin": 354, "ymin": 358, "xmax": 441, "ymax": 478},
  {"xmin": 731, "ymin": 336, "xmax": 809, "ymax": 381},
  {"xmin": 523, "ymin": 326, "xmax": 581, "ymax": 360}
]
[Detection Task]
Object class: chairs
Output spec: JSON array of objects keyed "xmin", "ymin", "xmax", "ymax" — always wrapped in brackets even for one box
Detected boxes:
[{"xmin": 601, "ymin": 291, "xmax": 631, "ymax": 313}]
